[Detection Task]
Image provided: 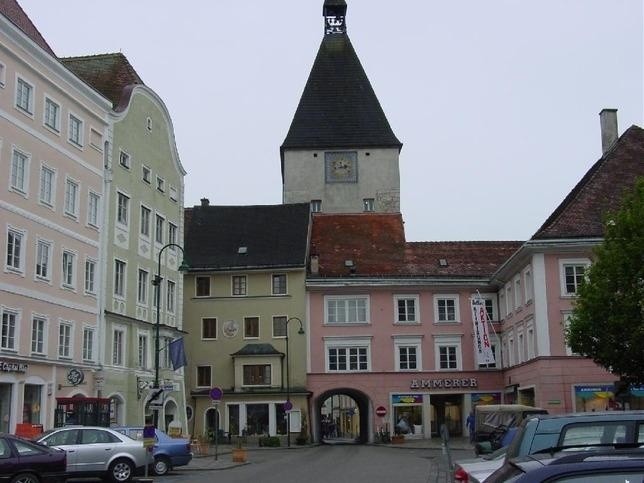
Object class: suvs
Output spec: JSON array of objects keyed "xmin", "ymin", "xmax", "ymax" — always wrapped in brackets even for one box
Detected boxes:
[
  {"xmin": 500, "ymin": 408, "xmax": 644, "ymax": 467},
  {"xmin": 473, "ymin": 402, "xmax": 549, "ymax": 451}
]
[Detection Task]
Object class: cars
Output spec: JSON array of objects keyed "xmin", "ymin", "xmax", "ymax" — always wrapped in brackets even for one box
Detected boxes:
[
  {"xmin": 28, "ymin": 424, "xmax": 154, "ymax": 482},
  {"xmin": 108, "ymin": 425, "xmax": 192, "ymax": 476},
  {"xmin": 0, "ymin": 430, "xmax": 68, "ymax": 482},
  {"xmin": 479, "ymin": 432, "xmax": 643, "ymax": 483},
  {"xmin": 447, "ymin": 443, "xmax": 509, "ymax": 482}
]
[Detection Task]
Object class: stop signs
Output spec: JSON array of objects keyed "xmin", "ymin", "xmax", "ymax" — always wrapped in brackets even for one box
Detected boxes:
[{"xmin": 374, "ymin": 405, "xmax": 387, "ymax": 418}]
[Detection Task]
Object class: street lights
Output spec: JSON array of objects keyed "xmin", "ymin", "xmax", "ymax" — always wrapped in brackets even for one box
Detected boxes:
[
  {"xmin": 151, "ymin": 241, "xmax": 192, "ymax": 427},
  {"xmin": 284, "ymin": 314, "xmax": 306, "ymax": 446}
]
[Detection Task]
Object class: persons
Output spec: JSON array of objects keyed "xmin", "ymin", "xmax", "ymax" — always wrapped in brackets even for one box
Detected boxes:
[{"xmin": 466, "ymin": 411, "xmax": 475, "ymax": 444}]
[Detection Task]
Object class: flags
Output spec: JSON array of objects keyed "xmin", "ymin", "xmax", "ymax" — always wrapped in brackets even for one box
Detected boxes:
[{"xmin": 169, "ymin": 337, "xmax": 187, "ymax": 370}]
